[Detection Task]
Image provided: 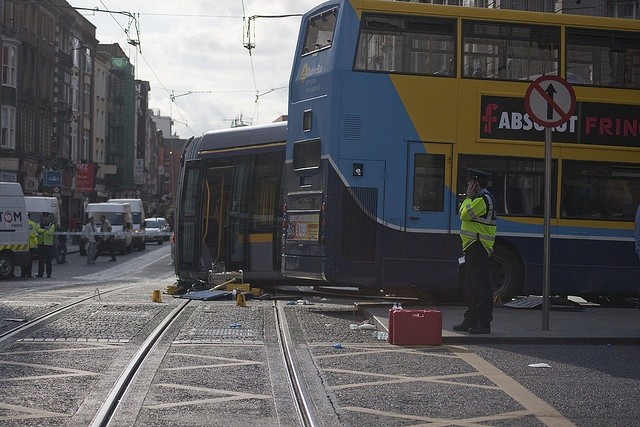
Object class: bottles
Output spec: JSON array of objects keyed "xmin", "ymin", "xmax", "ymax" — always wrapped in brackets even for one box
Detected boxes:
[
  {"xmin": 232, "ymin": 287, "xmax": 236, "ymax": 299},
  {"xmin": 392, "ymin": 302, "xmax": 397, "ymax": 309},
  {"xmin": 297, "ymin": 300, "xmax": 310, "ymax": 305},
  {"xmin": 371, "ymin": 331, "xmax": 388, "ymax": 341},
  {"xmin": 350, "ymin": 324, "xmax": 357, "ymax": 329},
  {"xmin": 397, "ymin": 303, "xmax": 403, "ymax": 310}
]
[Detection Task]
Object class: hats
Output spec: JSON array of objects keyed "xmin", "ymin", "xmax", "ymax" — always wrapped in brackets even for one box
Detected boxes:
[
  {"xmin": 42, "ymin": 211, "xmax": 51, "ymax": 216},
  {"xmin": 466, "ymin": 167, "xmax": 492, "ymax": 182}
]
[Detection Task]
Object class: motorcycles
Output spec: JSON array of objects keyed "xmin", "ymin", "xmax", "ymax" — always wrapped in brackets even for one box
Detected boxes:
[{"xmin": 72, "ymin": 224, "xmax": 81, "ymax": 244}]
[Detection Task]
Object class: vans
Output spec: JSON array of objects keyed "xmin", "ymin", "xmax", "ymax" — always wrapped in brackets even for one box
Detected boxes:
[
  {"xmin": 0, "ymin": 182, "xmax": 31, "ymax": 278},
  {"xmin": 106, "ymin": 198, "xmax": 147, "ymax": 251},
  {"xmin": 80, "ymin": 202, "xmax": 133, "ymax": 256},
  {"xmin": 24, "ymin": 195, "xmax": 61, "ymax": 260}
]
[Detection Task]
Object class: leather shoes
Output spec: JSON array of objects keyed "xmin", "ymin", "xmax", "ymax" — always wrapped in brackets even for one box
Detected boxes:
[
  {"xmin": 453, "ymin": 321, "xmax": 468, "ymax": 331},
  {"xmin": 467, "ymin": 323, "xmax": 491, "ymax": 333},
  {"xmin": 47, "ymin": 273, "xmax": 51, "ymax": 277},
  {"xmin": 36, "ymin": 273, "xmax": 43, "ymax": 277},
  {"xmin": 109, "ymin": 257, "xmax": 116, "ymax": 261}
]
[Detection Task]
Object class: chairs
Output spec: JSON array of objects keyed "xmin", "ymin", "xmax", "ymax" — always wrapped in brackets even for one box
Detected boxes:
[
  {"xmin": 414, "ymin": 56, "xmax": 637, "ymax": 89},
  {"xmin": 503, "ymin": 186, "xmax": 630, "ymax": 220}
]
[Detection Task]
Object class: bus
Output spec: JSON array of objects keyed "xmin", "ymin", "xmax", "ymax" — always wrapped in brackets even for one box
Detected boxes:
[
  {"xmin": 171, "ymin": 120, "xmax": 359, "ymax": 292},
  {"xmin": 281, "ymin": 0, "xmax": 638, "ymax": 307}
]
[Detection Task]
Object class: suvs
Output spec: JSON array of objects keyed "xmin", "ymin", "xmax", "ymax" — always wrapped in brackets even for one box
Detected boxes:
[
  {"xmin": 144, "ymin": 217, "xmax": 163, "ymax": 244},
  {"xmin": 156, "ymin": 217, "xmax": 171, "ymax": 240}
]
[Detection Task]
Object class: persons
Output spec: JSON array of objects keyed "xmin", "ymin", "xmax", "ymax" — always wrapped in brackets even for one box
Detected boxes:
[
  {"xmin": 58, "ymin": 216, "xmax": 68, "ymax": 264},
  {"xmin": 92, "ymin": 215, "xmax": 116, "ymax": 261},
  {"xmin": 84, "ymin": 217, "xmax": 97, "ymax": 264},
  {"xmin": 17, "ymin": 213, "xmax": 36, "ymax": 279},
  {"xmin": 452, "ymin": 168, "xmax": 497, "ymax": 334},
  {"xmin": 37, "ymin": 211, "xmax": 55, "ymax": 278}
]
